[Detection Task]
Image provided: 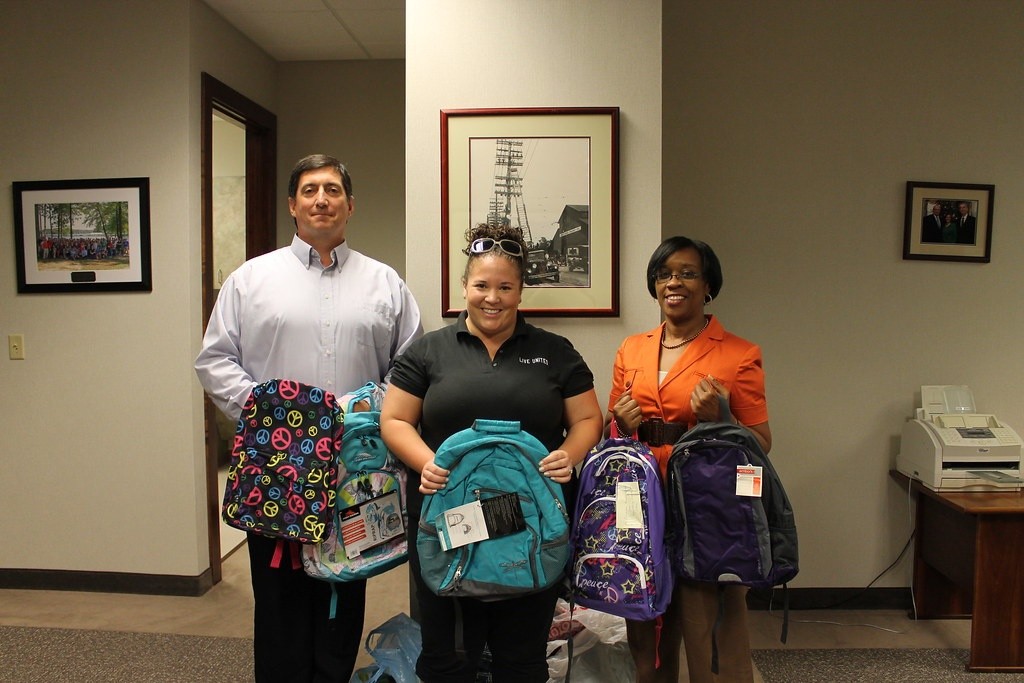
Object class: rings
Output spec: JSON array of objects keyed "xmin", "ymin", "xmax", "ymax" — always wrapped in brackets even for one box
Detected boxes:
[{"xmin": 569, "ymin": 468, "xmax": 573, "ymax": 474}]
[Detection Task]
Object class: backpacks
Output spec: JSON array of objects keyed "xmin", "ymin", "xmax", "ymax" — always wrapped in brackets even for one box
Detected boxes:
[
  {"xmin": 416, "ymin": 418, "xmax": 572, "ymax": 662},
  {"xmin": 302, "ymin": 381, "xmax": 409, "ymax": 619},
  {"xmin": 665, "ymin": 396, "xmax": 799, "ymax": 674},
  {"xmin": 559, "ymin": 417, "xmax": 674, "ymax": 683},
  {"xmin": 221, "ymin": 379, "xmax": 344, "ymax": 569}
]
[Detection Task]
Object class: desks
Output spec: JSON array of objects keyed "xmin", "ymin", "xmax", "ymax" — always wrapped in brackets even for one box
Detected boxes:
[{"xmin": 891, "ymin": 466, "xmax": 1024, "ymax": 674}]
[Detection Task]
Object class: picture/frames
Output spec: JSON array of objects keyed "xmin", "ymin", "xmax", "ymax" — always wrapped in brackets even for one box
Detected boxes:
[
  {"xmin": 12, "ymin": 177, "xmax": 153, "ymax": 294},
  {"xmin": 902, "ymin": 180, "xmax": 996, "ymax": 264},
  {"xmin": 439, "ymin": 106, "xmax": 620, "ymax": 318}
]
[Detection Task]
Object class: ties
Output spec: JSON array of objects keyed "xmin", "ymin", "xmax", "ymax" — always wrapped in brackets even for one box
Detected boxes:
[
  {"xmin": 962, "ymin": 218, "xmax": 964, "ymax": 226},
  {"xmin": 936, "ymin": 217, "xmax": 940, "ymax": 226}
]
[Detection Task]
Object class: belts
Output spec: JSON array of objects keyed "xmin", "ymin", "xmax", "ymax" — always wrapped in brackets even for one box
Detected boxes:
[{"xmin": 638, "ymin": 417, "xmax": 688, "ymax": 447}]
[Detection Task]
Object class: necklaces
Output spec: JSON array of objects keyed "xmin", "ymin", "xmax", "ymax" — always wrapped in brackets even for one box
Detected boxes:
[{"xmin": 661, "ymin": 319, "xmax": 709, "ymax": 349}]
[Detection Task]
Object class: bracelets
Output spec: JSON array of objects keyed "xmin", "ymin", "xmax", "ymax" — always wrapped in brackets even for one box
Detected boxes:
[
  {"xmin": 736, "ymin": 418, "xmax": 739, "ymax": 425},
  {"xmin": 616, "ymin": 423, "xmax": 629, "ymax": 438}
]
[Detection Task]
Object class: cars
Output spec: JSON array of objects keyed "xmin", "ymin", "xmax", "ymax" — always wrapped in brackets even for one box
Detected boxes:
[
  {"xmin": 567, "ymin": 245, "xmax": 589, "ymax": 273},
  {"xmin": 525, "ymin": 250, "xmax": 560, "ymax": 285}
]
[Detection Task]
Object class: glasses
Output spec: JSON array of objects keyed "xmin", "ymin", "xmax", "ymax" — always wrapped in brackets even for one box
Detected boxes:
[
  {"xmin": 469, "ymin": 238, "xmax": 523, "ymax": 260},
  {"xmin": 652, "ymin": 269, "xmax": 702, "ymax": 283}
]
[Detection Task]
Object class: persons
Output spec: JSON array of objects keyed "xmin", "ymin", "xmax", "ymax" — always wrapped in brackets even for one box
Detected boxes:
[
  {"xmin": 380, "ymin": 223, "xmax": 604, "ymax": 683},
  {"xmin": 38, "ymin": 236, "xmax": 129, "ymax": 260},
  {"xmin": 603, "ymin": 236, "xmax": 772, "ymax": 683},
  {"xmin": 922, "ymin": 201, "xmax": 977, "ymax": 244},
  {"xmin": 194, "ymin": 154, "xmax": 423, "ymax": 683}
]
